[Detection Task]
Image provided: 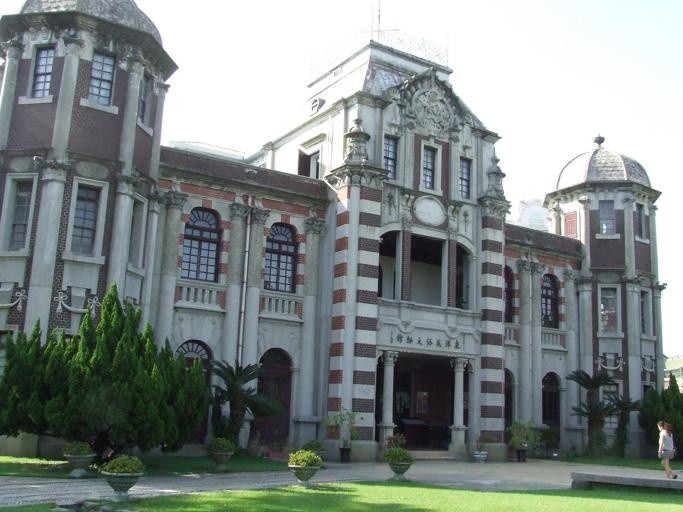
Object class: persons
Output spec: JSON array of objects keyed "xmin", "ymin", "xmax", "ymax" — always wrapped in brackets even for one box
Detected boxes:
[{"xmin": 655, "ymin": 420, "xmax": 678, "ymax": 480}]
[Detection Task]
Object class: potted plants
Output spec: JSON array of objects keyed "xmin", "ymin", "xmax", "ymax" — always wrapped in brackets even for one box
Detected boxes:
[
  {"xmin": 204, "ymin": 437, "xmax": 237, "ymax": 470},
  {"xmin": 300, "ymin": 441, "xmax": 327, "ymax": 458},
  {"xmin": 515, "ymin": 439, "xmax": 529, "ymax": 462},
  {"xmin": 286, "ymin": 449, "xmax": 323, "ymax": 485},
  {"xmin": 472, "ymin": 434, "xmax": 489, "ymax": 462},
  {"xmin": 382, "ymin": 446, "xmax": 416, "ymax": 481},
  {"xmin": 316, "ymin": 405, "xmax": 363, "ymax": 463},
  {"xmin": 88, "ymin": 454, "xmax": 146, "ymax": 497},
  {"xmin": 62, "ymin": 440, "xmax": 97, "ymax": 477}
]
